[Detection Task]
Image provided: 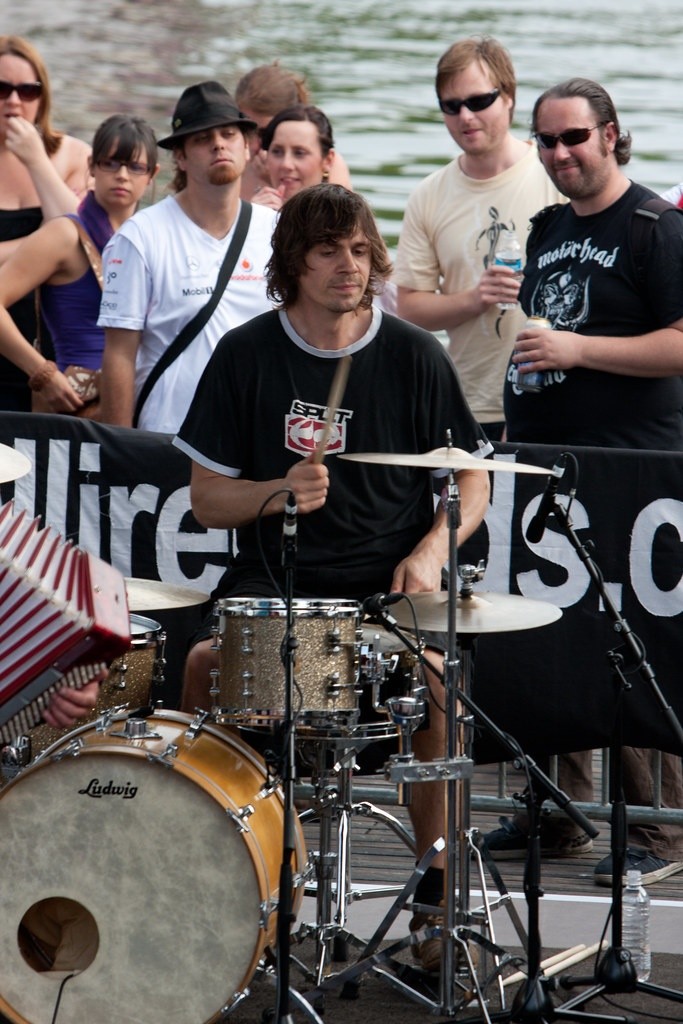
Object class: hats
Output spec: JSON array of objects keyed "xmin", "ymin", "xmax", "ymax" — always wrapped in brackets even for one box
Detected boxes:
[{"xmin": 157, "ymin": 80, "xmax": 258, "ymax": 150}]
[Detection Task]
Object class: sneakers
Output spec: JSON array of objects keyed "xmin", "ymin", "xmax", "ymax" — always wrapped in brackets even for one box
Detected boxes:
[
  {"xmin": 482, "ymin": 815, "xmax": 592, "ymax": 858},
  {"xmin": 593, "ymin": 846, "xmax": 683, "ymax": 885},
  {"xmin": 409, "ymin": 915, "xmax": 478, "ymax": 974}
]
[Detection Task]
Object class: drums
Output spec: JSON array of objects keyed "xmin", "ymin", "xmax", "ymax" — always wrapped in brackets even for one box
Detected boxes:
[
  {"xmin": 257, "ymin": 623, "xmax": 422, "ymax": 742},
  {"xmin": 1, "ymin": 615, "xmax": 166, "ymax": 768},
  {"xmin": 210, "ymin": 594, "xmax": 362, "ymax": 731},
  {"xmin": 1, "ymin": 710, "xmax": 312, "ymax": 1024}
]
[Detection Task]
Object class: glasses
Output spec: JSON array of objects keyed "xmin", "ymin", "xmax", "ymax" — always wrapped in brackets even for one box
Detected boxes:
[
  {"xmin": 97, "ymin": 157, "xmax": 150, "ymax": 174},
  {"xmin": 0, "ymin": 80, "xmax": 42, "ymax": 99},
  {"xmin": 438, "ymin": 88, "xmax": 500, "ymax": 114},
  {"xmin": 534, "ymin": 123, "xmax": 603, "ymax": 149}
]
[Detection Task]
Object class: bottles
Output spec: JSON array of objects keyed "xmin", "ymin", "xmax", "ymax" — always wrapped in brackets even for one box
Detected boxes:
[
  {"xmin": 494, "ymin": 229, "xmax": 522, "ymax": 310},
  {"xmin": 621, "ymin": 870, "xmax": 650, "ymax": 982}
]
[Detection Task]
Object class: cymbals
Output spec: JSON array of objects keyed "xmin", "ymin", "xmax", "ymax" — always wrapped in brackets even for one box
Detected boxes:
[
  {"xmin": 337, "ymin": 446, "xmax": 558, "ymax": 474},
  {"xmin": 127, "ymin": 575, "xmax": 215, "ymax": 612},
  {"xmin": 389, "ymin": 589, "xmax": 562, "ymax": 633},
  {"xmin": 0, "ymin": 442, "xmax": 33, "ymax": 485}
]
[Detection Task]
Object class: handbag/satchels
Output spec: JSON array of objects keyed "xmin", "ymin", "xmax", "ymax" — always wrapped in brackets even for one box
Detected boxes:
[{"xmin": 31, "ymin": 369, "xmax": 105, "ymax": 424}]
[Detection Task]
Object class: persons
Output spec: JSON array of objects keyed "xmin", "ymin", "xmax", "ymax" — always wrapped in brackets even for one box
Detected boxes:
[
  {"xmin": 0, "ymin": 114, "xmax": 160, "ymax": 421},
  {"xmin": 42, "ymin": 666, "xmax": 109, "ymax": 731},
  {"xmin": 94, "ymin": 81, "xmax": 286, "ymax": 433},
  {"xmin": 465, "ymin": 78, "xmax": 683, "ymax": 886},
  {"xmin": 0, "ymin": 34, "xmax": 99, "ymax": 418},
  {"xmin": 392, "ymin": 41, "xmax": 571, "ymax": 780},
  {"xmin": 173, "ymin": 182, "xmax": 483, "ymax": 980},
  {"xmin": 236, "ymin": 58, "xmax": 355, "ymax": 216}
]
